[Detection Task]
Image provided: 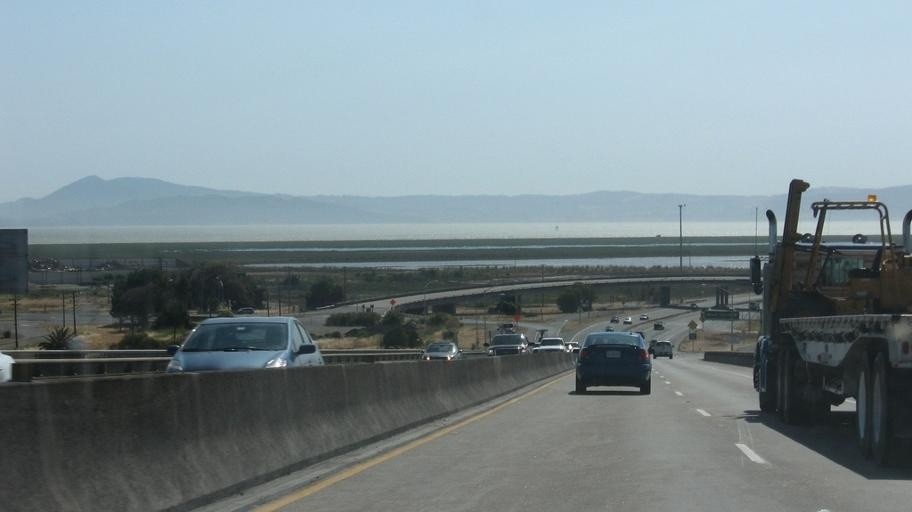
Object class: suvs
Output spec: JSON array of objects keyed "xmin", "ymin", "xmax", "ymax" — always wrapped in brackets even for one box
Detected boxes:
[{"xmin": 748, "ymin": 302, "xmax": 760, "ymax": 312}]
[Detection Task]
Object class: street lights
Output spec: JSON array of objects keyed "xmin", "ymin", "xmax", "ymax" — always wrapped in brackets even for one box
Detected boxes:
[{"xmin": 677, "ymin": 203, "xmax": 686, "ymax": 296}]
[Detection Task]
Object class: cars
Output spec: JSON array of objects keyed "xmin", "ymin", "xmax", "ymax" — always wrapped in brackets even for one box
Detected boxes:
[
  {"xmin": 483, "ymin": 313, "xmax": 674, "ymax": 359},
  {"xmin": 572, "ymin": 331, "xmax": 655, "ymax": 394},
  {"xmin": 420, "ymin": 342, "xmax": 463, "ymax": 361},
  {"xmin": 689, "ymin": 303, "xmax": 697, "ymax": 310},
  {"xmin": 166, "ymin": 316, "xmax": 325, "ymax": 373},
  {"xmin": 0, "ymin": 352, "xmax": 32, "ymax": 385},
  {"xmin": 235, "ymin": 306, "xmax": 256, "ymax": 315}
]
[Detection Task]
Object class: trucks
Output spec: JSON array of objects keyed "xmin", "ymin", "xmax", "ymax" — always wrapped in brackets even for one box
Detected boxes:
[{"xmin": 749, "ymin": 179, "xmax": 911, "ymax": 466}]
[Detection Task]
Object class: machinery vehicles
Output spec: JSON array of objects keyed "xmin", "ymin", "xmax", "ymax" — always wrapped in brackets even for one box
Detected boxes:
[{"xmin": 769, "ymin": 179, "xmax": 911, "ymax": 337}]
